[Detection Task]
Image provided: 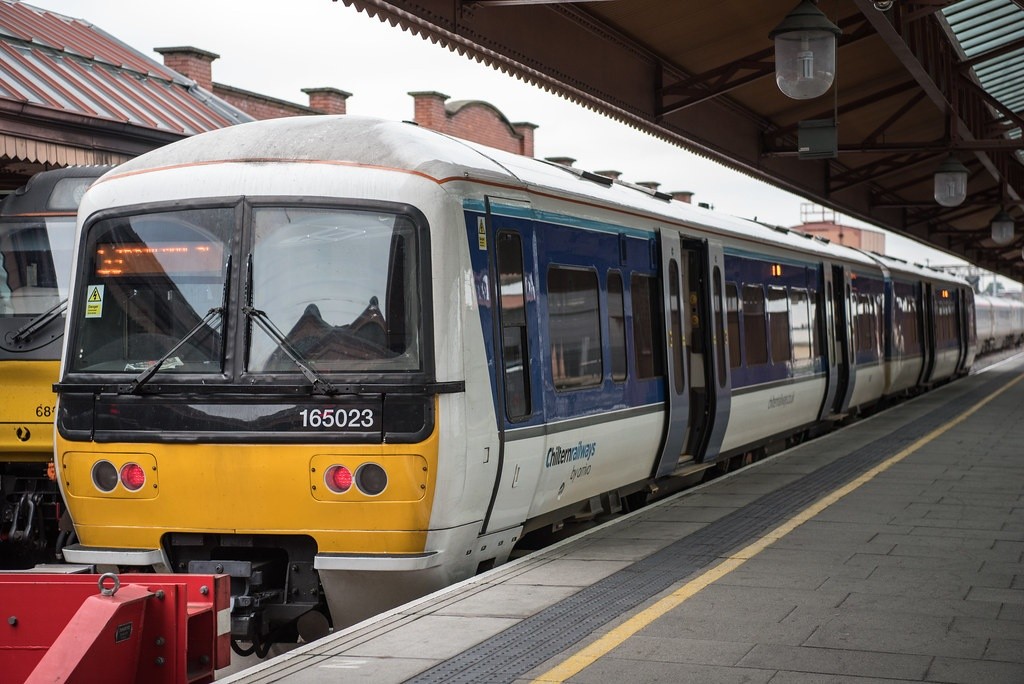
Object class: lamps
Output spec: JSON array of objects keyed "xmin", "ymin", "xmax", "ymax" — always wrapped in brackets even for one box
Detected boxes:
[
  {"xmin": 989, "ymin": 205, "xmax": 1016, "ymax": 245},
  {"xmin": 930, "ymin": 148, "xmax": 971, "ymax": 209},
  {"xmin": 768, "ymin": 1, "xmax": 841, "ymax": 100}
]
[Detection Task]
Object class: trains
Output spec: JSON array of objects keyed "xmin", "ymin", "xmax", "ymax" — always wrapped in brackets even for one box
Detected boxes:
[
  {"xmin": 56, "ymin": 114, "xmax": 976, "ymax": 671},
  {"xmin": 0, "ymin": 167, "xmax": 120, "ymax": 569},
  {"xmin": 974, "ymin": 296, "xmax": 1022, "ymax": 357}
]
